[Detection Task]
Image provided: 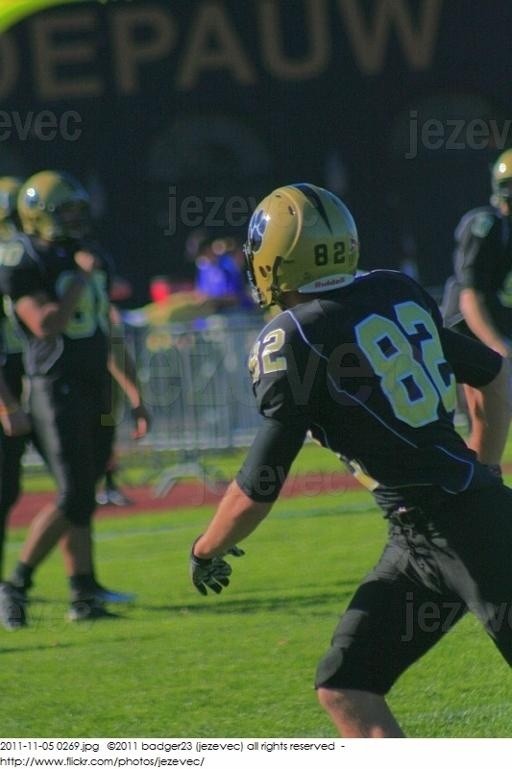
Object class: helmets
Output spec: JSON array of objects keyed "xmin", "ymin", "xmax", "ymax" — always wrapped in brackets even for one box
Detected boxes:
[
  {"xmin": 489, "ymin": 148, "xmax": 512, "ymax": 217},
  {"xmin": 0, "ymin": 167, "xmax": 91, "ymax": 242},
  {"xmin": 241, "ymin": 182, "xmax": 360, "ymax": 309}
]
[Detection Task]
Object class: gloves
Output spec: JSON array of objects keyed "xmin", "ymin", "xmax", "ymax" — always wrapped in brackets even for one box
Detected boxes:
[{"xmin": 190, "ymin": 535, "xmax": 244, "ymax": 595}]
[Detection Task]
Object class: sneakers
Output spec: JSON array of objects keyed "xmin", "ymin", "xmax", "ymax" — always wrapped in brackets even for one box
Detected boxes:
[
  {"xmin": 67, "ymin": 588, "xmax": 137, "ymax": 621},
  {"xmin": 0, "ymin": 580, "xmax": 29, "ymax": 630},
  {"xmin": 96, "ymin": 485, "xmax": 134, "ymax": 510}
]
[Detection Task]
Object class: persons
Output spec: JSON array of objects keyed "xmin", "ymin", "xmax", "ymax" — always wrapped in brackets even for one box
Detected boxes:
[
  {"xmin": 436, "ymin": 148, "xmax": 510, "ymax": 467},
  {"xmin": 187, "ymin": 179, "xmax": 510, "ymax": 737},
  {"xmin": 183, "ymin": 232, "xmax": 261, "ymax": 433},
  {"xmin": 0, "ymin": 169, "xmax": 151, "ymax": 629},
  {"xmin": 0, "ymin": 176, "xmax": 51, "ymax": 563},
  {"xmin": 92, "ymin": 352, "xmax": 134, "ymax": 508}
]
